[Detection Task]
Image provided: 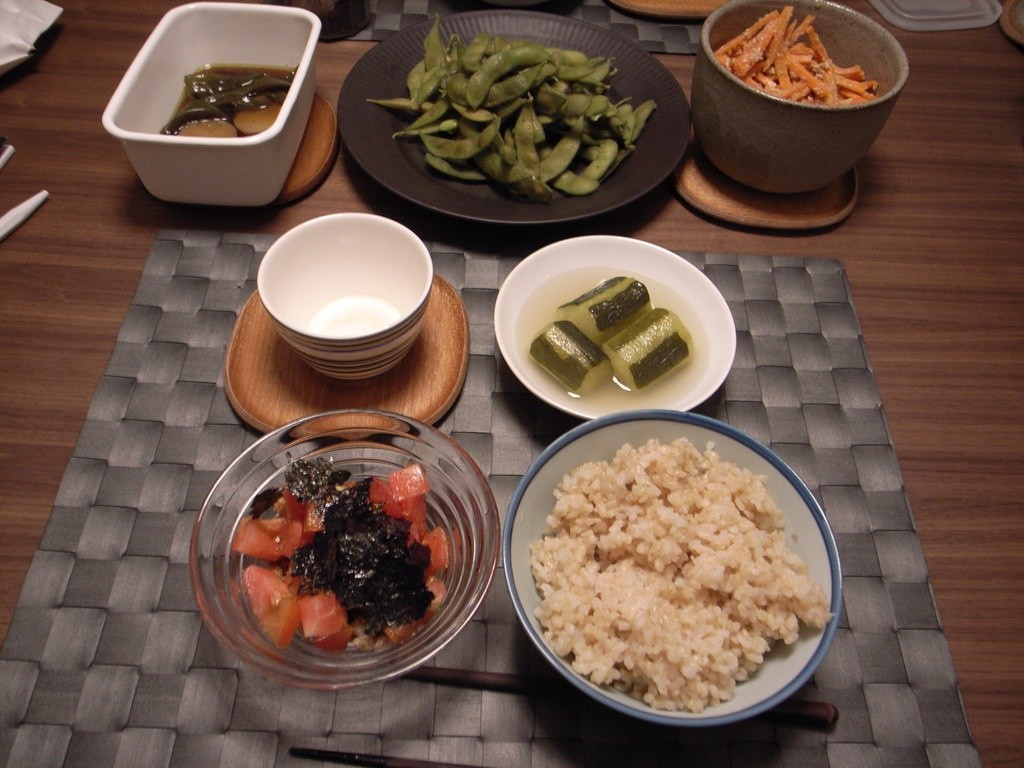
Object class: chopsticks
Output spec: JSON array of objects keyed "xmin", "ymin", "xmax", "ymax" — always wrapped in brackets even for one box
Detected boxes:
[{"xmin": 288, "ymin": 665, "xmax": 838, "ymax": 768}]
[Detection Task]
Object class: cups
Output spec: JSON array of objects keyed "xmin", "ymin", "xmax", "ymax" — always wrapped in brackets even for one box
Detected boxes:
[{"xmin": 289, "ymin": 0, "xmax": 370, "ymax": 40}]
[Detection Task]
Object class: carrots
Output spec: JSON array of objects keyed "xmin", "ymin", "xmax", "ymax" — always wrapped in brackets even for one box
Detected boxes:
[{"xmin": 713, "ymin": 4, "xmax": 878, "ymax": 107}]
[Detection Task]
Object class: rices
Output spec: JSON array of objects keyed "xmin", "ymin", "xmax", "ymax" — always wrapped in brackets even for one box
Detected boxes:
[{"xmin": 530, "ymin": 438, "xmax": 835, "ymax": 713}]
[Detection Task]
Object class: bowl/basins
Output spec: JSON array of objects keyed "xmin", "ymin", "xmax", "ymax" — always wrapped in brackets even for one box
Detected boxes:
[
  {"xmin": 691, "ymin": 0, "xmax": 909, "ymax": 192},
  {"xmin": 102, "ymin": 2, "xmax": 322, "ymax": 206},
  {"xmin": 258, "ymin": 212, "xmax": 434, "ymax": 379},
  {"xmin": 189, "ymin": 408, "xmax": 502, "ymax": 689},
  {"xmin": 502, "ymin": 410, "xmax": 843, "ymax": 726}
]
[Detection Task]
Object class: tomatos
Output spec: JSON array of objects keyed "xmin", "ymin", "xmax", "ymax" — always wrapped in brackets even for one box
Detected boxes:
[{"xmin": 234, "ymin": 463, "xmax": 451, "ymax": 651}]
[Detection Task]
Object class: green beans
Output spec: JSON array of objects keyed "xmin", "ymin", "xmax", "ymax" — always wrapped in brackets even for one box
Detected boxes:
[{"xmin": 364, "ymin": 13, "xmax": 657, "ymax": 200}]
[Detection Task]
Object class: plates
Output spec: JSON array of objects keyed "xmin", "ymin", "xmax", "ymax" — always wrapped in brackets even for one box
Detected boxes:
[
  {"xmin": 491, "ymin": 235, "xmax": 737, "ymax": 420},
  {"xmin": 337, "ymin": 9, "xmax": 690, "ymax": 224}
]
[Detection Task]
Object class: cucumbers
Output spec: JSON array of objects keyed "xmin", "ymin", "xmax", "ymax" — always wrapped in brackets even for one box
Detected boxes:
[{"xmin": 526, "ymin": 276, "xmax": 693, "ymax": 396}]
